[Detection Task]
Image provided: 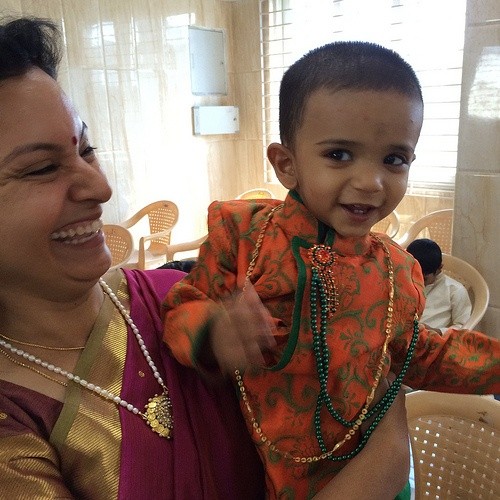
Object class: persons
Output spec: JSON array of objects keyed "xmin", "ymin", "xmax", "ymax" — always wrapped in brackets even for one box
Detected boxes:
[
  {"xmin": 158, "ymin": 41, "xmax": 500, "ymax": 500},
  {"xmin": 405, "ymin": 239, "xmax": 472, "ymax": 335},
  {"xmin": 0, "ymin": 13, "xmax": 410, "ymax": 500}
]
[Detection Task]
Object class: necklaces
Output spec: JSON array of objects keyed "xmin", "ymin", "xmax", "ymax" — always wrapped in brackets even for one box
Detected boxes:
[
  {"xmin": 231, "ymin": 203, "xmax": 394, "ymax": 463},
  {"xmin": 0, "ymin": 276, "xmax": 174, "ymax": 441}
]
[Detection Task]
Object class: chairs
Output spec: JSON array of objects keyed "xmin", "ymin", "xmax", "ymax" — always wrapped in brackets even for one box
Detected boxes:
[
  {"xmin": 370, "ymin": 209, "xmax": 401, "ymax": 239},
  {"xmin": 437, "ymin": 253, "xmax": 491, "ymax": 335},
  {"xmin": 401, "ymin": 388, "xmax": 500, "ymax": 500},
  {"xmin": 98, "ymin": 224, "xmax": 134, "ymax": 281},
  {"xmin": 114, "ymin": 199, "xmax": 180, "ymax": 268},
  {"xmin": 392, "ymin": 208, "xmax": 454, "ymax": 257},
  {"xmin": 165, "ymin": 234, "xmax": 209, "ymax": 264},
  {"xmin": 234, "ymin": 189, "xmax": 274, "ymax": 200}
]
